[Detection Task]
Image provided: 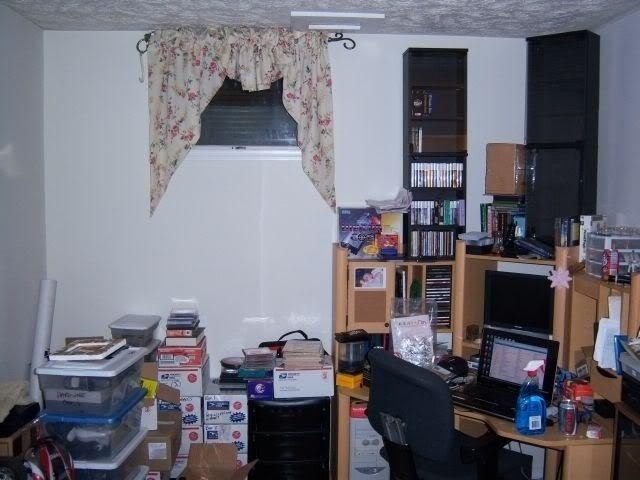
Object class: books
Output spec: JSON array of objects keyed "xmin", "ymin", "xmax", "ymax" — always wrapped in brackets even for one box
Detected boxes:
[
  {"xmin": 411, "ymin": 199, "xmax": 465, "ymax": 226},
  {"xmin": 412, "ymin": 231, "xmax": 455, "ymax": 258},
  {"xmin": 479, "ymin": 193, "xmax": 526, "ymax": 240},
  {"xmin": 161, "ymin": 312, "xmax": 205, "ymax": 346},
  {"xmin": 283, "ymin": 338, "xmax": 326, "ymax": 370},
  {"xmin": 411, "ymin": 86, "xmax": 434, "ymax": 120},
  {"xmin": 411, "ymin": 159, "xmax": 466, "ymax": 188},
  {"xmin": 408, "ymin": 127, "xmax": 424, "ymax": 154},
  {"xmin": 552, "ymin": 210, "xmax": 606, "ymax": 266}
]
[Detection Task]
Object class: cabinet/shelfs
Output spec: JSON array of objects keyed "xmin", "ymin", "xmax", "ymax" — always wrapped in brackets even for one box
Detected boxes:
[
  {"xmin": 402, "ymin": 47, "xmax": 469, "ymax": 261},
  {"xmin": 333, "ymin": 241, "xmax": 640, "ymax": 366},
  {"xmin": 2, "ymin": 416, "xmax": 41, "ymax": 460},
  {"xmin": 524, "ymin": 29, "xmax": 601, "ymax": 253},
  {"xmin": 582, "ymin": 234, "xmax": 640, "ymax": 282}
]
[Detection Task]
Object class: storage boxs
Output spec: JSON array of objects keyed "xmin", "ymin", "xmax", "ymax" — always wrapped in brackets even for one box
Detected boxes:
[
  {"xmin": 130, "ymin": 465, "xmax": 150, "ymax": 480},
  {"xmin": 32, "ymin": 344, "xmax": 152, "ymax": 420},
  {"xmin": 104, "ymin": 312, "xmax": 163, "ymax": 345},
  {"xmin": 483, "ymin": 142, "xmax": 524, "ymax": 196},
  {"xmin": 581, "ymin": 345, "xmax": 621, "ymax": 403},
  {"xmin": 42, "ymin": 388, "xmax": 152, "ymax": 463},
  {"xmin": 164, "ymin": 354, "xmax": 212, "ymax": 480},
  {"xmin": 274, "ymin": 354, "xmax": 335, "ymax": 398},
  {"xmin": 130, "ymin": 337, "xmax": 160, "ymax": 361},
  {"xmin": 202, "ymin": 380, "xmax": 249, "ymax": 465}
]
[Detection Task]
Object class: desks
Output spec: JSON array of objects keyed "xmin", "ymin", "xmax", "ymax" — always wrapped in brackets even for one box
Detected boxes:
[{"xmin": 336, "ymin": 377, "xmax": 616, "ymax": 479}]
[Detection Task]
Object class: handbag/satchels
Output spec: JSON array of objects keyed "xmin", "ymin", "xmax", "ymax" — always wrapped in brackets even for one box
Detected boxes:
[{"xmin": 259, "ymin": 339, "xmax": 328, "ymax": 359}]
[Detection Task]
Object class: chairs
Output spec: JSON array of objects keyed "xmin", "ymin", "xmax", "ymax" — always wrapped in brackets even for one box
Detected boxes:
[{"xmin": 363, "ymin": 353, "xmax": 535, "ymax": 477}]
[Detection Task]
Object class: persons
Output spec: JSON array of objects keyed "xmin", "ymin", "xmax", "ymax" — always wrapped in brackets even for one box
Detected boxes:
[{"xmin": 358, "ymin": 270, "xmax": 383, "ymax": 287}]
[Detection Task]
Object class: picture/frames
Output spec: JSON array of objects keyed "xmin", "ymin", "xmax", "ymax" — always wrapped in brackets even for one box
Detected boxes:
[
  {"xmin": 49, "ymin": 337, "xmax": 127, "ymax": 363},
  {"xmin": 355, "ymin": 268, "xmax": 387, "ymax": 292}
]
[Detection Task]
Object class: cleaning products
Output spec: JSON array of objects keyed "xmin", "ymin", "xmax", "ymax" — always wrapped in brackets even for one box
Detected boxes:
[{"xmin": 514, "ymin": 359, "xmax": 548, "ymax": 438}]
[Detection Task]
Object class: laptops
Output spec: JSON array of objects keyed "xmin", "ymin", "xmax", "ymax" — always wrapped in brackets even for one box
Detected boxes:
[{"xmin": 449, "ymin": 328, "xmax": 560, "ymax": 422}]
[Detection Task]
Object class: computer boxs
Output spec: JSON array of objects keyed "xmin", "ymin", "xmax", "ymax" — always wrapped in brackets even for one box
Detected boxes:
[{"xmin": 349, "ymin": 417, "xmax": 390, "ymax": 480}]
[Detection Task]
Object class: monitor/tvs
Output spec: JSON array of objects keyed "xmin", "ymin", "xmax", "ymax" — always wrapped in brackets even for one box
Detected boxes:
[{"xmin": 483, "ymin": 270, "xmax": 555, "ymax": 334}]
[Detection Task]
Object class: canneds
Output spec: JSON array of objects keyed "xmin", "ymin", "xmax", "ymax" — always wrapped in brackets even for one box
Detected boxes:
[
  {"xmin": 559, "ymin": 400, "xmax": 578, "ymax": 436},
  {"xmin": 576, "ymin": 395, "xmax": 593, "ymax": 427},
  {"xmin": 601, "ymin": 249, "xmax": 619, "ymax": 282}
]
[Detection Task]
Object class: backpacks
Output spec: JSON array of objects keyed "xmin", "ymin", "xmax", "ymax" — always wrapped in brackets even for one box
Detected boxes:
[{"xmin": 23, "ymin": 439, "xmax": 76, "ymax": 480}]
